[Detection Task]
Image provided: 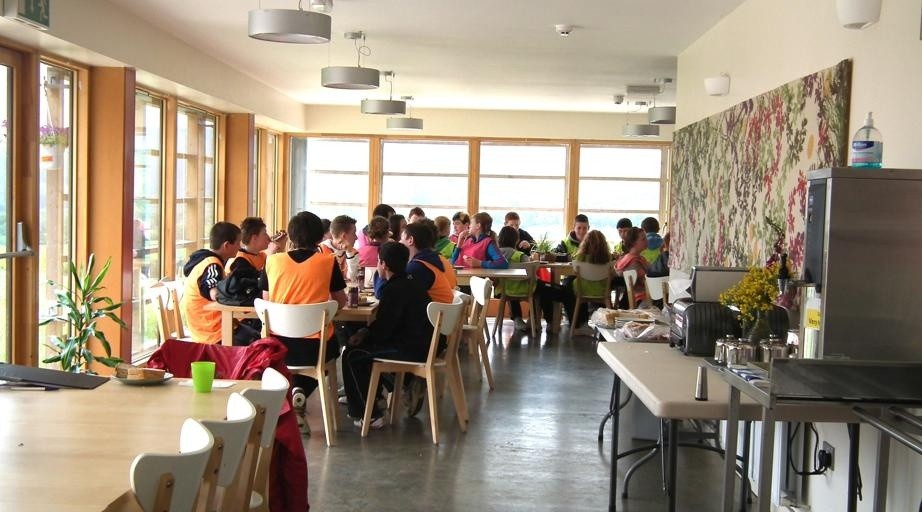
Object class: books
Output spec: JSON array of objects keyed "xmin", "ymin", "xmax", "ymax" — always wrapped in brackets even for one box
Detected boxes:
[{"xmin": 9, "ymin": 385, "xmax": 49, "ymax": 391}]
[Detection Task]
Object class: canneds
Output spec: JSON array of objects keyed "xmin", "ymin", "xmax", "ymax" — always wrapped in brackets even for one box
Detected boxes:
[
  {"xmin": 758, "ymin": 333, "xmax": 791, "ymax": 363},
  {"xmin": 714, "ymin": 336, "xmax": 756, "ymax": 366}
]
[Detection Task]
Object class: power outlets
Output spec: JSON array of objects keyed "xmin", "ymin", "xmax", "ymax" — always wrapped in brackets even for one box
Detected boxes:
[{"xmin": 821, "ymin": 441, "xmax": 835, "ymax": 471}]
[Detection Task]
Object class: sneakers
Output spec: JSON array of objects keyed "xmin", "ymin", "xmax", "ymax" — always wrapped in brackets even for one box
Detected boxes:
[
  {"xmin": 406, "ymin": 377, "xmax": 426, "ymax": 418},
  {"xmin": 337, "ymin": 382, "xmax": 388, "ymax": 430},
  {"xmin": 575, "ymin": 325, "xmax": 594, "ymax": 336},
  {"xmin": 292, "ymin": 387, "xmax": 312, "ymax": 434},
  {"xmin": 514, "ymin": 319, "xmax": 542, "ymax": 334}
]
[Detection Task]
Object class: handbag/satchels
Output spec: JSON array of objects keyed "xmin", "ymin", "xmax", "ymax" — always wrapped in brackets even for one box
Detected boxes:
[{"xmin": 216, "ymin": 266, "xmax": 261, "ymax": 306}]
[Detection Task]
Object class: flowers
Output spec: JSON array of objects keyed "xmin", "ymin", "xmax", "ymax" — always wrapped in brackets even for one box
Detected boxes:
[{"xmin": 718, "ymin": 262, "xmax": 783, "ymax": 329}]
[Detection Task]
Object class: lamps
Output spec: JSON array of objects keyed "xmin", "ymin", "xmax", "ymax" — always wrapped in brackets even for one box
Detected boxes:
[
  {"xmin": 704, "ymin": 72, "xmax": 730, "ymax": 97},
  {"xmin": 836, "ymin": 0, "xmax": 881, "ymax": 30},
  {"xmin": 360, "ymin": 71, "xmax": 405, "ymax": 115},
  {"xmin": 321, "ymin": 31, "xmax": 380, "ymax": 90},
  {"xmin": 386, "ymin": 96, "xmax": 422, "ymax": 130},
  {"xmin": 626, "ymin": 85, "xmax": 661, "ymax": 96},
  {"xmin": 248, "ymin": 0, "xmax": 332, "ymax": 43},
  {"xmin": 623, "ymin": 101, "xmax": 659, "ymax": 135},
  {"xmin": 648, "ymin": 92, "xmax": 676, "ymax": 124}
]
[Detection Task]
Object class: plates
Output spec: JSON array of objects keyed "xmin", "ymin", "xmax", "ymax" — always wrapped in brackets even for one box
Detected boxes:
[
  {"xmin": 453, "ymin": 265, "xmax": 465, "ymax": 269},
  {"xmin": 111, "ymin": 373, "xmax": 174, "ymax": 385},
  {"xmin": 359, "ymin": 301, "xmax": 375, "ymax": 306}
]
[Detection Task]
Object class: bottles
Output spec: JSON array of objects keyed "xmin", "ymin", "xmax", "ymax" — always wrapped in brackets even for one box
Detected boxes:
[
  {"xmin": 269, "ymin": 231, "xmax": 286, "ymax": 242},
  {"xmin": 695, "ymin": 366, "xmax": 709, "ymax": 401},
  {"xmin": 715, "ymin": 333, "xmax": 755, "ymax": 366},
  {"xmin": 759, "ymin": 334, "xmax": 787, "ymax": 361}
]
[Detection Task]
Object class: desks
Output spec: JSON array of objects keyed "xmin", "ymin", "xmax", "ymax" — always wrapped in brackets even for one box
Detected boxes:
[{"xmin": 596, "ymin": 341, "xmax": 905, "ymax": 512}]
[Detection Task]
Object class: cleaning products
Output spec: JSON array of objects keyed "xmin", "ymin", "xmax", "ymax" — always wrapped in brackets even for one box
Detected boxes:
[{"xmin": 851, "ymin": 109, "xmax": 884, "ymax": 169}]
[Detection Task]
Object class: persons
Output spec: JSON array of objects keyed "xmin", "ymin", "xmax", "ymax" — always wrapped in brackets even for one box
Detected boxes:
[
  {"xmin": 179, "ymin": 201, "xmax": 509, "ymax": 434},
  {"xmin": 495, "ymin": 209, "xmax": 670, "ymax": 335}
]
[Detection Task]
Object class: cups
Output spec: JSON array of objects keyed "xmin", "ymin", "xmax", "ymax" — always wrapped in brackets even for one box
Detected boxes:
[{"xmin": 192, "ymin": 362, "xmax": 215, "ymax": 393}]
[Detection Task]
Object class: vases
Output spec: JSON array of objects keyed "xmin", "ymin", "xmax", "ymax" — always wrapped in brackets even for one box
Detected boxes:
[{"xmin": 749, "ymin": 309, "xmax": 776, "ymax": 362}]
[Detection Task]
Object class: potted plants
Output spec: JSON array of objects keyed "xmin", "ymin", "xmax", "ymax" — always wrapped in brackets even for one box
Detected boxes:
[
  {"xmin": 613, "ymin": 242, "xmax": 625, "ymax": 262},
  {"xmin": 535, "ymin": 232, "xmax": 550, "ymax": 261}
]
